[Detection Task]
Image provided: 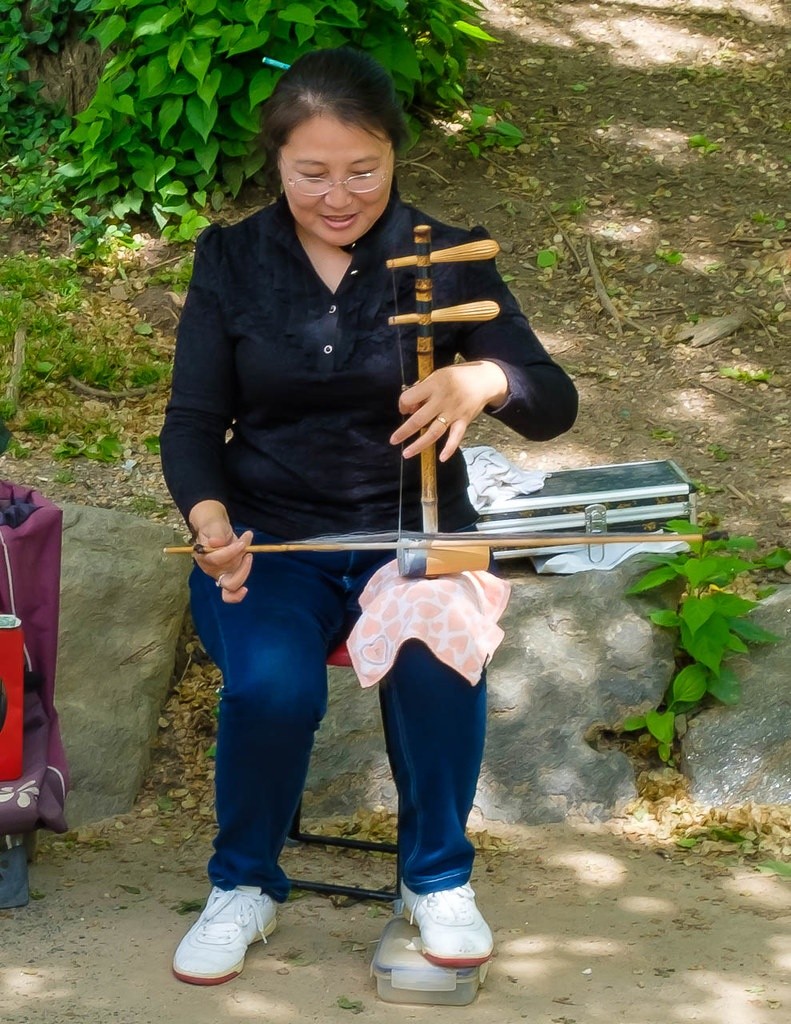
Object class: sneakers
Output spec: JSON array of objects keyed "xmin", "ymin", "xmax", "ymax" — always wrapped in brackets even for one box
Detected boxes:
[
  {"xmin": 400, "ymin": 877, "xmax": 494, "ymax": 967},
  {"xmin": 173, "ymin": 884, "xmax": 277, "ymax": 986}
]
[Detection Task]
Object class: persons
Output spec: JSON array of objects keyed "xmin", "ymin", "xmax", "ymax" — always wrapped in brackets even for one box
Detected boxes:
[{"xmin": 158, "ymin": 52, "xmax": 579, "ymax": 985}]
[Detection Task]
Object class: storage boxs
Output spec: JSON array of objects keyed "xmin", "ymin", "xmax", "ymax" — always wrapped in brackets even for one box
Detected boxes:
[{"xmin": 476, "ymin": 459, "xmax": 696, "ymax": 560}]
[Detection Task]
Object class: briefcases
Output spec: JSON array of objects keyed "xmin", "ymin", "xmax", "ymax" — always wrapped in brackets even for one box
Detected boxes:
[{"xmin": 476, "ymin": 457, "xmax": 698, "ymax": 558}]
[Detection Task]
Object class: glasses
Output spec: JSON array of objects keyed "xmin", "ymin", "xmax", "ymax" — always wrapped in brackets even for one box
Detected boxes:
[{"xmin": 278, "ymin": 140, "xmax": 393, "ymax": 197}]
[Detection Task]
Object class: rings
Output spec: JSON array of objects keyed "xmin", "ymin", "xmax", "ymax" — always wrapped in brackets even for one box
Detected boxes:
[
  {"xmin": 215, "ymin": 574, "xmax": 226, "ymax": 587},
  {"xmin": 436, "ymin": 416, "xmax": 450, "ymax": 428}
]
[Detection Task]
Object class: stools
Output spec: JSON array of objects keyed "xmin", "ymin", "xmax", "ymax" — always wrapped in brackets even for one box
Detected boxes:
[{"xmin": 288, "ymin": 641, "xmax": 400, "ymax": 902}]
